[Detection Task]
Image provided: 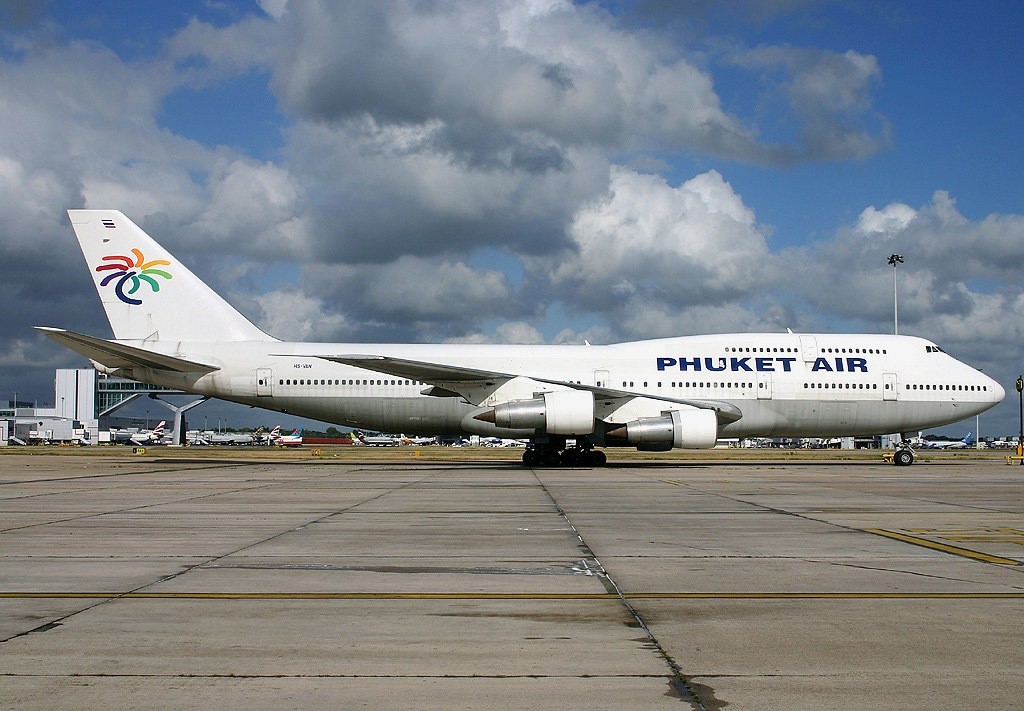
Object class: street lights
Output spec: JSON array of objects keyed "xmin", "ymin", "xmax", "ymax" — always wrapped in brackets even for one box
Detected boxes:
[{"xmin": 887, "ymin": 253, "xmax": 904, "ymax": 335}]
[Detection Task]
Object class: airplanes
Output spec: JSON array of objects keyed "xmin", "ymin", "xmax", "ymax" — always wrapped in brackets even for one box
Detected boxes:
[
  {"xmin": 107, "ymin": 419, "xmax": 535, "ymax": 449},
  {"xmin": 729, "ymin": 424, "xmax": 1023, "ymax": 449},
  {"xmin": 32, "ymin": 209, "xmax": 1005, "ymax": 471}
]
